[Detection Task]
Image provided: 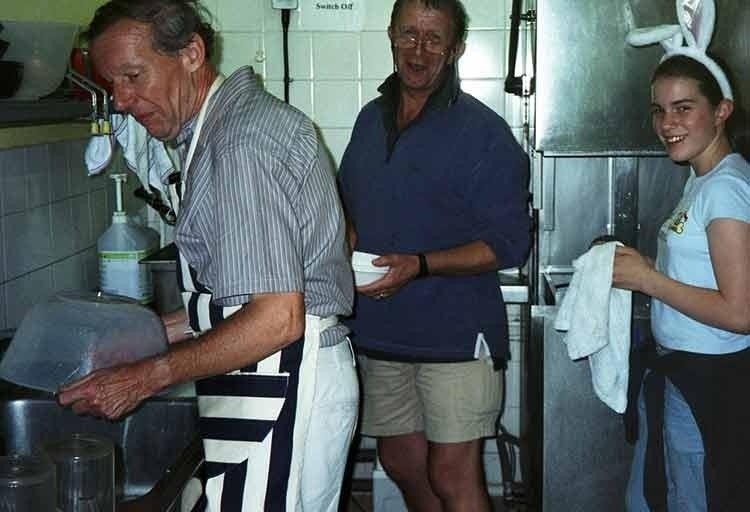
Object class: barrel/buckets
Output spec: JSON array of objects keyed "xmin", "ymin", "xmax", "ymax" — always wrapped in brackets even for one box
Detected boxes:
[{"xmin": 96, "ymin": 167, "xmax": 163, "ymax": 306}]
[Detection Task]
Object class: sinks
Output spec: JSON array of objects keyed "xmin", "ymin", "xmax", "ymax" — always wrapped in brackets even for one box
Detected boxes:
[{"xmin": 138, "ymin": 243, "xmax": 186, "ymax": 312}]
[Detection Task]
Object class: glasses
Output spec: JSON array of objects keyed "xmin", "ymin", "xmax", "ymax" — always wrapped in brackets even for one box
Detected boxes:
[{"xmin": 393, "ymin": 32, "xmax": 449, "ymax": 57}]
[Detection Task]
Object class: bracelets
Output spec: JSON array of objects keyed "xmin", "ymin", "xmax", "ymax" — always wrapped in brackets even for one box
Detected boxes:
[{"xmin": 415, "ymin": 253, "xmax": 437, "ymax": 280}]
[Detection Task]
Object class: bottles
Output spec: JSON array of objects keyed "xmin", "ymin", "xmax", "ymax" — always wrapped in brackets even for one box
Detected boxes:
[{"xmin": 0, "ymin": 433, "xmax": 115, "ymax": 511}]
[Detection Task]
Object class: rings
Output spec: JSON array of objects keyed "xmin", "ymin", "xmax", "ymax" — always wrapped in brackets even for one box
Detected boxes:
[{"xmin": 375, "ymin": 292, "xmax": 385, "ymax": 302}]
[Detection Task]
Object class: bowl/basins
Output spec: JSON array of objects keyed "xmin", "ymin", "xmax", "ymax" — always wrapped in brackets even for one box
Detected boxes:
[
  {"xmin": 349, "ymin": 248, "xmax": 390, "ymax": 287},
  {"xmin": 1, "ymin": 289, "xmax": 172, "ymax": 397},
  {"xmin": 0, "ymin": 18, "xmax": 78, "ymax": 102}
]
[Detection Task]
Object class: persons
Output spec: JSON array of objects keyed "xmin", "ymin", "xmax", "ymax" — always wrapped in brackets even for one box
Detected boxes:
[
  {"xmin": 337, "ymin": 0, "xmax": 532, "ymax": 512},
  {"xmin": 57, "ymin": 0, "xmax": 361, "ymax": 512},
  {"xmin": 612, "ymin": 56, "xmax": 750, "ymax": 512}
]
